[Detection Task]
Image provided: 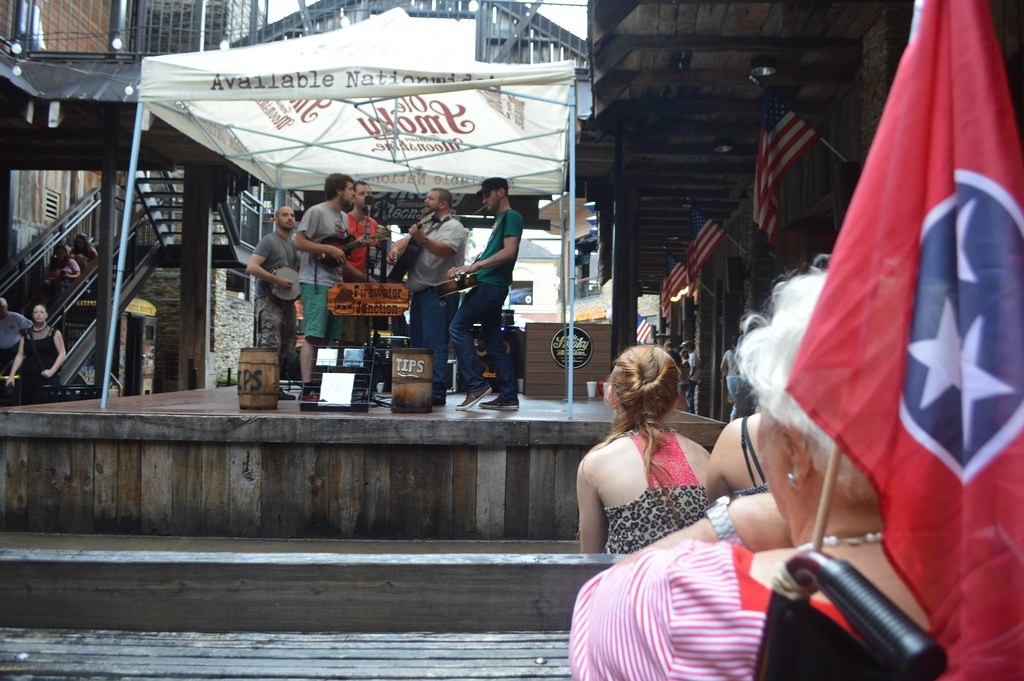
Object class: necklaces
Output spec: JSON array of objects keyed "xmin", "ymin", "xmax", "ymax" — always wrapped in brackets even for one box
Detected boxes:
[{"xmin": 820, "ymin": 530, "xmax": 886, "ymax": 546}]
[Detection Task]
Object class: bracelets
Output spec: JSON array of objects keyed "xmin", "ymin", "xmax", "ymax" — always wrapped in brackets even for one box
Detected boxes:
[{"xmin": 421, "ymin": 238, "xmax": 428, "ymax": 246}]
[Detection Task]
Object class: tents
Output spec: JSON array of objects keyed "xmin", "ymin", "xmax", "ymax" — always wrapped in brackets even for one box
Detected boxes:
[{"xmin": 101, "ymin": 11, "xmax": 579, "ymax": 417}]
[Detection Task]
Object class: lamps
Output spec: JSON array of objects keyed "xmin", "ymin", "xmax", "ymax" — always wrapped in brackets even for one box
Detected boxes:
[
  {"xmin": 750, "ymin": 56, "xmax": 776, "ymax": 76},
  {"xmin": 713, "ymin": 137, "xmax": 733, "ymax": 153}
]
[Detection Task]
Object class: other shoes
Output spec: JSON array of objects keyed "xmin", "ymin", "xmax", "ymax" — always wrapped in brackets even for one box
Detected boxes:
[
  {"xmin": 432, "ymin": 396, "xmax": 446, "ymax": 406},
  {"xmin": 277, "ymin": 388, "xmax": 295, "ymax": 401}
]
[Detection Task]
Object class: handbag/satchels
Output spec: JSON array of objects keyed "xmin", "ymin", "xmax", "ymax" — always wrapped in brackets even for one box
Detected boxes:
[{"xmin": 39, "ymin": 376, "xmax": 66, "ymax": 403}]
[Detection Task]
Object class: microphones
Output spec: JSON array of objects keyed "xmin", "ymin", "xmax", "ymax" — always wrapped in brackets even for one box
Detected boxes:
[{"xmin": 365, "ymin": 195, "xmax": 374, "ymax": 217}]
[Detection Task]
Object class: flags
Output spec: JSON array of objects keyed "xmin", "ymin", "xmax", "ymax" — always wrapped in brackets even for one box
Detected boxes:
[
  {"xmin": 636, "ymin": 249, "xmax": 696, "ymax": 345},
  {"xmin": 684, "ymin": 199, "xmax": 728, "ymax": 286},
  {"xmin": 752, "ymin": 86, "xmax": 821, "ymax": 249},
  {"xmin": 788, "ymin": 0, "xmax": 1023, "ymax": 680}
]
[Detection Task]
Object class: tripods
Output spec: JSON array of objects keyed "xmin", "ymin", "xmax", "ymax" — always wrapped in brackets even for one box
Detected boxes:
[{"xmin": 351, "ymin": 217, "xmax": 393, "ymax": 410}]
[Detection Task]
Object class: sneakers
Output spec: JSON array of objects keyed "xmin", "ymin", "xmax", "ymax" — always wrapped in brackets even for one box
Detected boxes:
[
  {"xmin": 478, "ymin": 399, "xmax": 520, "ymax": 412},
  {"xmin": 456, "ymin": 385, "xmax": 493, "ymax": 411},
  {"xmin": 298, "ymin": 391, "xmax": 319, "ymax": 403}
]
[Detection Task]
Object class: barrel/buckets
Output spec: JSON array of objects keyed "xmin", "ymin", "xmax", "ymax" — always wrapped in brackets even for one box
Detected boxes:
[
  {"xmin": 391, "ymin": 348, "xmax": 433, "ymax": 412},
  {"xmin": 237, "ymin": 346, "xmax": 279, "ymax": 410}
]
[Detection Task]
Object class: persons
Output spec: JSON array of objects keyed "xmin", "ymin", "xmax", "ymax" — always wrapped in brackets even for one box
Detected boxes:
[
  {"xmin": 704, "ymin": 412, "xmax": 773, "ymax": 506},
  {"xmin": 659, "ymin": 253, "xmax": 832, "ymax": 422},
  {"xmin": 293, "ymin": 173, "xmax": 356, "ymax": 403},
  {"xmin": 447, "ymin": 177, "xmax": 524, "ymax": 412},
  {"xmin": 386, "ymin": 188, "xmax": 468, "ymax": 406},
  {"xmin": 567, "ymin": 265, "xmax": 930, "ymax": 681},
  {"xmin": 69, "ymin": 233, "xmax": 99, "ymax": 287},
  {"xmin": 0, "ymin": 297, "xmax": 33, "ymax": 376},
  {"xmin": 4, "ymin": 301, "xmax": 67, "ymax": 406},
  {"xmin": 39, "ymin": 244, "xmax": 81, "ymax": 305},
  {"xmin": 247, "ymin": 205, "xmax": 303, "ymax": 401},
  {"xmin": 337, "ymin": 180, "xmax": 378, "ymax": 365},
  {"xmin": 576, "ymin": 345, "xmax": 712, "ymax": 554}
]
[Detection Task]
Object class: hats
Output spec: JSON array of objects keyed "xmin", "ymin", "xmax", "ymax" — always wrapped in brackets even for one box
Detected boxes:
[
  {"xmin": 477, "ymin": 178, "xmax": 509, "ymax": 196},
  {"xmin": 679, "ymin": 342, "xmax": 694, "ymax": 350}
]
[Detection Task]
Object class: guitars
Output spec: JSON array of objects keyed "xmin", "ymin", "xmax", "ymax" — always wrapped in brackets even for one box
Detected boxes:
[
  {"xmin": 266, "ymin": 264, "xmax": 301, "ymax": 303},
  {"xmin": 313, "ymin": 227, "xmax": 392, "ymax": 267},
  {"xmin": 387, "ymin": 211, "xmax": 441, "ymax": 282}
]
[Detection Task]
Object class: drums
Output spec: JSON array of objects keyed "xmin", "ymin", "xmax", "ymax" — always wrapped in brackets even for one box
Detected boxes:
[{"xmin": 434, "ymin": 273, "xmax": 480, "ymax": 298}]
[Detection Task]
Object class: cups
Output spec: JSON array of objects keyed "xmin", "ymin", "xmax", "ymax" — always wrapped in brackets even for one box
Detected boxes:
[
  {"xmin": 603, "ymin": 383, "xmax": 608, "ymax": 394},
  {"xmin": 377, "ymin": 382, "xmax": 385, "ymax": 394},
  {"xmin": 517, "ymin": 378, "xmax": 524, "ymax": 393},
  {"xmin": 587, "ymin": 381, "xmax": 596, "ymax": 397},
  {"xmin": 598, "ymin": 380, "xmax": 604, "ymax": 395}
]
